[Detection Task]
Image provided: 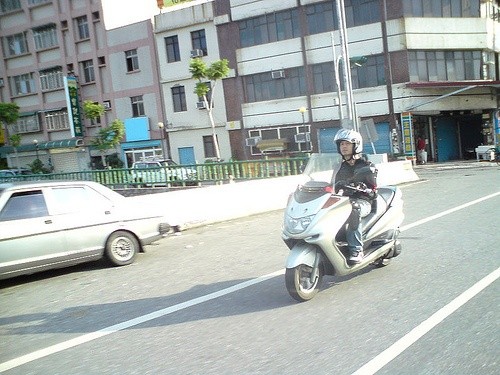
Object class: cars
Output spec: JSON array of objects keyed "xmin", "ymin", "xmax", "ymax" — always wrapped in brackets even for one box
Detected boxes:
[
  {"xmin": 0, "ymin": 169, "xmax": 25, "ymax": 182},
  {"xmin": 131, "ymin": 158, "xmax": 196, "ymax": 189},
  {"xmin": 0, "ymin": 179, "xmax": 173, "ymax": 289}
]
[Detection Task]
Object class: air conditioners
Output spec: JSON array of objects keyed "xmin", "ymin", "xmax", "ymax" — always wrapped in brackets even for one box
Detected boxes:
[{"xmin": 103, "ymin": 102, "xmax": 111, "ymax": 108}]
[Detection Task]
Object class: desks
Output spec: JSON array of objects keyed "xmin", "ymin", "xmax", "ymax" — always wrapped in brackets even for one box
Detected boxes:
[{"xmin": 476, "ymin": 152, "xmax": 495, "ymax": 162}]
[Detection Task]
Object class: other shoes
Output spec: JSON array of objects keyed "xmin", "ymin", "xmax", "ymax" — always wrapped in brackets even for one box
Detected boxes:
[{"xmin": 348, "ymin": 251, "xmax": 364, "ymax": 265}]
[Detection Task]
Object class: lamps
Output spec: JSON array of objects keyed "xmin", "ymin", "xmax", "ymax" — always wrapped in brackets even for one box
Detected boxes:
[
  {"xmin": 272, "ymin": 71, "xmax": 285, "ymax": 79},
  {"xmin": 294, "ymin": 133, "xmax": 310, "ymax": 143},
  {"xmin": 246, "ymin": 138, "xmax": 259, "ymax": 146},
  {"xmin": 196, "ymin": 101, "xmax": 205, "ymax": 108},
  {"xmin": 190, "ymin": 49, "xmax": 203, "ymax": 57}
]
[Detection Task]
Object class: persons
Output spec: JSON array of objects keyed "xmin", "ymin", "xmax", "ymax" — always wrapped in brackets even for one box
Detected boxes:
[
  {"xmin": 417, "ymin": 135, "xmax": 426, "ymax": 165},
  {"xmin": 329, "ymin": 129, "xmax": 378, "ymax": 263}
]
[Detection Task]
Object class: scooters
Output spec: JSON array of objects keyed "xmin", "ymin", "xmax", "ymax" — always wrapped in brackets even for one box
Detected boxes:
[{"xmin": 278, "ymin": 152, "xmax": 409, "ymax": 305}]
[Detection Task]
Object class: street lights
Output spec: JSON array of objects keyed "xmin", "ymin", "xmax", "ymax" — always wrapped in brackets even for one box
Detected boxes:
[
  {"xmin": 33, "ymin": 139, "xmax": 40, "ymax": 160},
  {"xmin": 158, "ymin": 122, "xmax": 166, "ymax": 159},
  {"xmin": 298, "ymin": 106, "xmax": 310, "ymax": 157}
]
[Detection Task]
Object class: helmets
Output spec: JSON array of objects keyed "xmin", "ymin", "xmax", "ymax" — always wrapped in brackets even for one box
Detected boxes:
[{"xmin": 335, "ymin": 130, "xmax": 362, "ymax": 153}]
